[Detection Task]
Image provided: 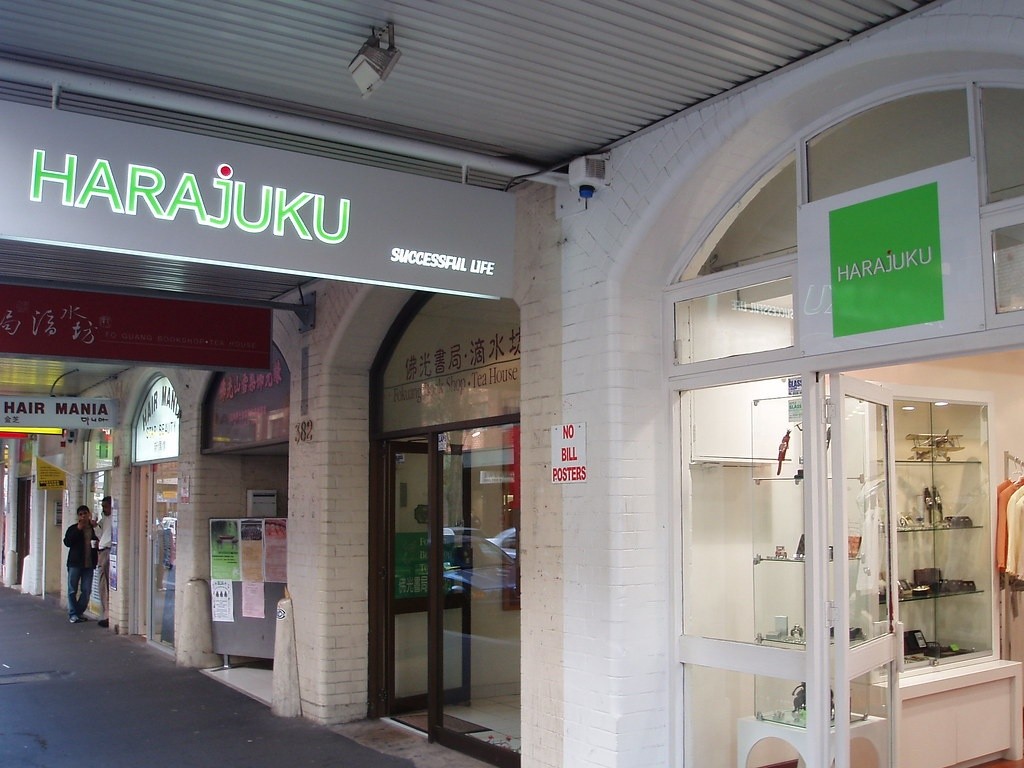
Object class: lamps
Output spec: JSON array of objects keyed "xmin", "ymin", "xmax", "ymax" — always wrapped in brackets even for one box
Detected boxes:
[{"xmin": 348, "ymin": 21, "xmax": 404, "ymax": 100}]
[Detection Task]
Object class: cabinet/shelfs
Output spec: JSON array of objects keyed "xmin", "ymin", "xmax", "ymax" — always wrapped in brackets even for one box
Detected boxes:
[
  {"xmin": 850, "ymin": 385, "xmax": 999, "ymax": 686},
  {"xmin": 850, "ymin": 660, "xmax": 1023, "ymax": 767},
  {"xmin": 691, "ymin": 306, "xmax": 794, "ymax": 468},
  {"xmin": 748, "ymin": 395, "xmax": 873, "ymax": 726}
]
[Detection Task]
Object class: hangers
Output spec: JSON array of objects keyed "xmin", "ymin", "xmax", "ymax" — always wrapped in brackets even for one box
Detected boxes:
[{"xmin": 1006, "ymin": 457, "xmax": 1024, "ymax": 483}]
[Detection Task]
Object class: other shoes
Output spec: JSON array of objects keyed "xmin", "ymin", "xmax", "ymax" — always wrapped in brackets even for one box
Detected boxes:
[
  {"xmin": 78, "ymin": 615, "xmax": 88, "ymax": 622},
  {"xmin": 70, "ymin": 616, "xmax": 79, "ymax": 623},
  {"xmin": 97, "ymin": 618, "xmax": 109, "ymax": 628}
]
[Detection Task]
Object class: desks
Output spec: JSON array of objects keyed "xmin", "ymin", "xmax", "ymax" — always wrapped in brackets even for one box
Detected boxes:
[{"xmin": 734, "ymin": 708, "xmax": 887, "ymax": 768}]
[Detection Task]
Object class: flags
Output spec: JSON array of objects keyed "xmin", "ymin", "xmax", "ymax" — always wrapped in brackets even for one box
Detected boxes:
[{"xmin": 37, "ymin": 456, "xmax": 66, "ymax": 490}]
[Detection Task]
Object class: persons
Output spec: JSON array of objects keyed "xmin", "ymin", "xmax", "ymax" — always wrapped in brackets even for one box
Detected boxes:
[
  {"xmin": 470, "ymin": 512, "xmax": 481, "ymax": 529},
  {"xmin": 63, "ymin": 496, "xmax": 111, "ymax": 626}
]
